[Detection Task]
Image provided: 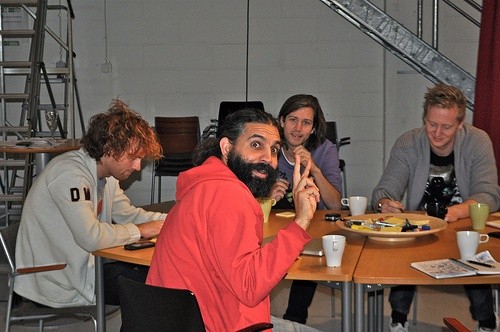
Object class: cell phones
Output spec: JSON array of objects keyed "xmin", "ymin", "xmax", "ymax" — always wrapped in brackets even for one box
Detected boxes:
[
  {"xmin": 124, "ymin": 241, "xmax": 155, "ymax": 250},
  {"xmin": 326, "ymin": 213, "xmax": 341, "ymax": 221}
]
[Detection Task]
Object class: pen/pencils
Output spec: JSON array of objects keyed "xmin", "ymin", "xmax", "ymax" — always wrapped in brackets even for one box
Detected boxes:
[
  {"xmin": 467, "ymin": 260, "xmax": 495, "ymax": 268},
  {"xmin": 384, "ymin": 191, "xmax": 405, "ymax": 213}
]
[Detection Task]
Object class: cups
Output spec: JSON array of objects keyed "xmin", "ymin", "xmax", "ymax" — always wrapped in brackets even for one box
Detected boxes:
[
  {"xmin": 255, "ymin": 198, "xmax": 276, "ymax": 223},
  {"xmin": 422, "ymin": 201, "xmax": 448, "ymax": 220},
  {"xmin": 322, "ymin": 235, "xmax": 346, "ymax": 267},
  {"xmin": 469, "ymin": 204, "xmax": 490, "ymax": 230},
  {"xmin": 456, "ymin": 230, "xmax": 489, "ymax": 260},
  {"xmin": 341, "ymin": 196, "xmax": 368, "ymax": 216}
]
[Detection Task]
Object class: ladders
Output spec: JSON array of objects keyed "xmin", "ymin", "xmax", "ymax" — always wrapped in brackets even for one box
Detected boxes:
[
  {"xmin": 0, "ymin": 0, "xmax": 47, "ymax": 216},
  {"xmin": 6, "ymin": 0, "xmax": 86, "ymax": 209}
]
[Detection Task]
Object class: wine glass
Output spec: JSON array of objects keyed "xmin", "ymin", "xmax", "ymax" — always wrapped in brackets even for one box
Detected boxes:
[{"xmin": 45, "ymin": 108, "xmax": 58, "ymax": 144}]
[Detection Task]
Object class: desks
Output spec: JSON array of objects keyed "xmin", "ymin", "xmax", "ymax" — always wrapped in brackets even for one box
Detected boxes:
[
  {"xmin": 0, "ymin": 138, "xmax": 84, "ymax": 180},
  {"xmin": 91, "ymin": 209, "xmax": 500, "ymax": 332}
]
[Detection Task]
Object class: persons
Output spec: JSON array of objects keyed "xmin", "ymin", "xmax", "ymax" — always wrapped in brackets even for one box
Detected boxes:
[
  {"xmin": 8, "ymin": 100, "xmax": 168, "ymax": 306},
  {"xmin": 269, "ymin": 94, "xmax": 341, "ymax": 326},
  {"xmin": 146, "ymin": 108, "xmax": 324, "ymax": 332},
  {"xmin": 372, "ymin": 84, "xmax": 500, "ymax": 332}
]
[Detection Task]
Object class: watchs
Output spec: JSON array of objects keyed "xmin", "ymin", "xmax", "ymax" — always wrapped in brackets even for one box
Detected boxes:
[{"xmin": 376, "ymin": 202, "xmax": 384, "ymax": 212}]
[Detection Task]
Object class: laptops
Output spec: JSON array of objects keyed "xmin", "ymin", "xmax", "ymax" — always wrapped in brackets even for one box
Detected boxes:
[{"xmin": 259, "ymin": 234, "xmax": 327, "ymax": 257}]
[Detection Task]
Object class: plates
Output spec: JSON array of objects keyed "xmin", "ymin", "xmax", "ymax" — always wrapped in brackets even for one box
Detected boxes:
[{"xmin": 335, "ymin": 213, "xmax": 447, "ymax": 242}]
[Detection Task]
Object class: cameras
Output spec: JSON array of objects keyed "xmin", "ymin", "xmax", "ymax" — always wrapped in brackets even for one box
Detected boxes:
[{"xmin": 425, "ymin": 197, "xmax": 461, "ymax": 219}]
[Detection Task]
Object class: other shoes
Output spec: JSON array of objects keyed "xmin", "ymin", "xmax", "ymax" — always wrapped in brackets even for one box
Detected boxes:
[
  {"xmin": 388, "ymin": 312, "xmax": 409, "ymax": 332},
  {"xmin": 476, "ymin": 320, "xmax": 497, "ymax": 332}
]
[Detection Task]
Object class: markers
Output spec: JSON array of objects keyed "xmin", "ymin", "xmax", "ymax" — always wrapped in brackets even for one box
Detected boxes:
[
  {"xmin": 353, "ymin": 221, "xmax": 374, "ymax": 228},
  {"xmin": 418, "ymin": 225, "xmax": 430, "ymax": 230}
]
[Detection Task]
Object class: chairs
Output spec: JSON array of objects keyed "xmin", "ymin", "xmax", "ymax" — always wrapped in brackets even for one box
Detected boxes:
[
  {"xmin": 0, "ymin": 220, "xmax": 120, "ymax": 332},
  {"xmin": 151, "ymin": 100, "xmax": 350, "ymax": 205},
  {"xmin": 117, "ymin": 276, "xmax": 273, "ymax": 332}
]
